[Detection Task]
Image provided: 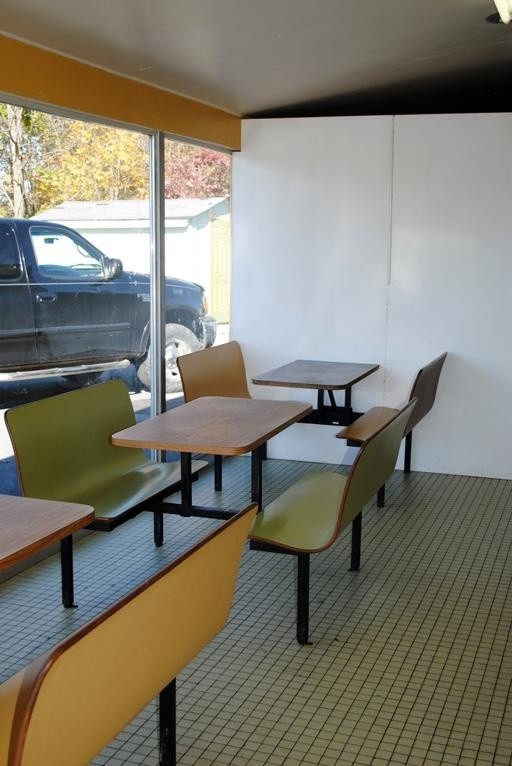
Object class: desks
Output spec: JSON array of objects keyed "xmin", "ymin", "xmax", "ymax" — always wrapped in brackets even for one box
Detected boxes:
[
  {"xmin": 110, "ymin": 394, "xmax": 314, "ymax": 522},
  {"xmin": 176, "ymin": 340, "xmax": 267, "ymax": 490},
  {"xmin": 0, "ymin": 492, "xmax": 97, "ymax": 576},
  {"xmin": 252, "ymin": 359, "xmax": 380, "ymax": 428}
]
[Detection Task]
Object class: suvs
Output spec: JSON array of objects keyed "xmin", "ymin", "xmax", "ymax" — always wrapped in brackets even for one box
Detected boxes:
[{"xmin": 0, "ymin": 216, "xmax": 216, "ymax": 392}]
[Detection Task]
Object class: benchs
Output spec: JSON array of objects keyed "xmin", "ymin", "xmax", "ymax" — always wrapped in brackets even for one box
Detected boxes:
[
  {"xmin": 0, "ymin": 501, "xmax": 259, "ymax": 766},
  {"xmin": 245, "ymin": 396, "xmax": 418, "ymax": 646},
  {"xmin": 335, "ymin": 349, "xmax": 448, "ymax": 508},
  {"xmin": 4, "ymin": 379, "xmax": 211, "ymax": 609}
]
